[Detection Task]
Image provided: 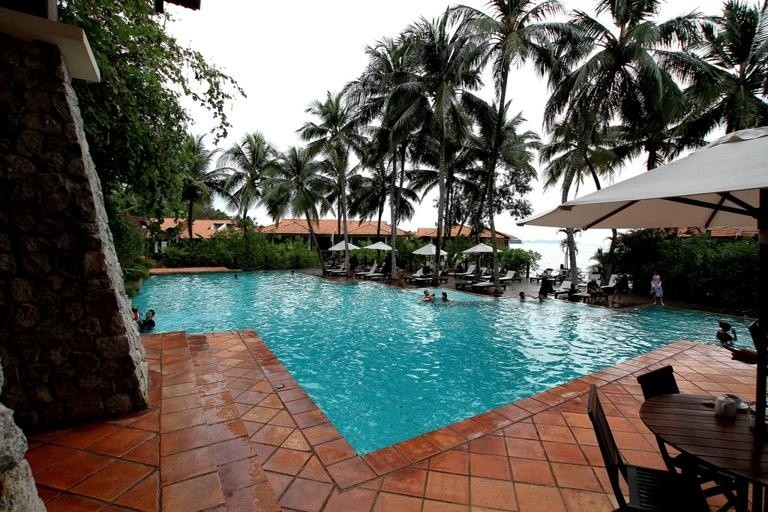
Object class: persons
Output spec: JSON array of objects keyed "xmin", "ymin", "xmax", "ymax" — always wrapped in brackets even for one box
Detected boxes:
[
  {"xmin": 586, "ymin": 276, "xmax": 604, "ymax": 302},
  {"xmin": 136, "ymin": 309, "xmax": 155, "ymax": 332},
  {"xmin": 441, "ymin": 292, "xmax": 450, "ymax": 302},
  {"xmin": 456, "ymin": 262, "xmax": 463, "ymax": 273},
  {"xmin": 427, "ymin": 293, "xmax": 435, "ymax": 299},
  {"xmin": 348, "ymin": 253, "xmax": 360, "ymax": 278},
  {"xmin": 549, "ymin": 264, "xmax": 566, "ymax": 280},
  {"xmin": 131, "ymin": 306, "xmax": 140, "ymax": 321},
  {"xmin": 535, "ymin": 270, "xmax": 549, "ymax": 278},
  {"xmin": 379, "ymin": 252, "xmax": 392, "ymax": 275},
  {"xmin": 611, "ymin": 274, "xmax": 632, "ymax": 305},
  {"xmin": 722, "ymin": 344, "xmax": 757, "ymax": 366},
  {"xmin": 519, "ymin": 291, "xmax": 525, "ymax": 301},
  {"xmin": 470, "ymin": 265, "xmax": 506, "ymax": 278},
  {"xmin": 716, "ymin": 320, "xmax": 737, "ymax": 345},
  {"xmin": 612, "ymin": 273, "xmax": 622, "ymax": 284},
  {"xmin": 538, "ymin": 294, "xmax": 544, "ymax": 303},
  {"xmin": 650, "ymin": 270, "xmax": 665, "ymax": 306},
  {"xmin": 289, "ymin": 269, "xmax": 295, "ymax": 276},
  {"xmin": 422, "ymin": 289, "xmax": 434, "ymax": 301},
  {"xmin": 234, "ymin": 273, "xmax": 239, "ymax": 279}
]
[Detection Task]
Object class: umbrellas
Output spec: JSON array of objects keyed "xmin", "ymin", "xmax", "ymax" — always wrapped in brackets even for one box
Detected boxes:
[
  {"xmin": 412, "ymin": 244, "xmax": 448, "ymax": 274},
  {"xmin": 328, "ymin": 240, "xmax": 361, "ymax": 268},
  {"xmin": 462, "ymin": 243, "xmax": 504, "ymax": 270},
  {"xmin": 514, "ymin": 123, "xmax": 768, "ymax": 512},
  {"xmin": 363, "ymin": 241, "xmax": 398, "ymax": 252}
]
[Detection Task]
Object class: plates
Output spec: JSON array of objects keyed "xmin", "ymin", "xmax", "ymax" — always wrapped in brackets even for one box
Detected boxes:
[{"xmin": 718, "ymin": 396, "xmax": 749, "ymax": 410}]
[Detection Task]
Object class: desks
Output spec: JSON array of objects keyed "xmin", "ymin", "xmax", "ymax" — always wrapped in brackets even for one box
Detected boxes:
[{"xmin": 639, "ymin": 393, "xmax": 768, "ymax": 512}]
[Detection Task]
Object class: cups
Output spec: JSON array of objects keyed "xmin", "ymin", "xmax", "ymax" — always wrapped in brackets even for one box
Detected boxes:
[{"xmin": 723, "ymin": 394, "xmax": 742, "ymax": 405}]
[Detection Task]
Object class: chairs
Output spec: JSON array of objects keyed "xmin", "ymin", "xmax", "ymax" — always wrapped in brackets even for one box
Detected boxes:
[
  {"xmin": 637, "ymin": 365, "xmax": 750, "ymax": 512},
  {"xmin": 323, "ymin": 258, "xmax": 517, "ymax": 289},
  {"xmin": 528, "ymin": 268, "xmax": 627, "ymax": 304},
  {"xmin": 589, "ymin": 384, "xmax": 713, "ymax": 512}
]
